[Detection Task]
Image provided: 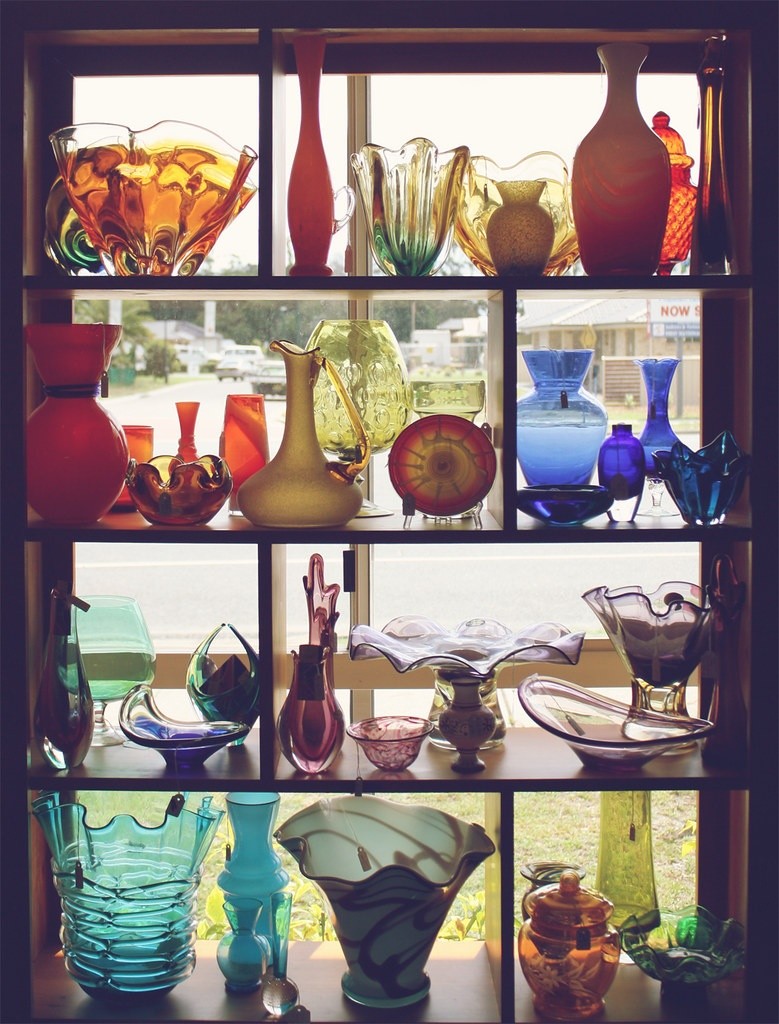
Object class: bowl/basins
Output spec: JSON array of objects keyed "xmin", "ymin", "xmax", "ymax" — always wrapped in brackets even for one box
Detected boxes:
[
  {"xmin": 346, "ymin": 715, "xmax": 434, "ymax": 773},
  {"xmin": 514, "ymin": 483, "xmax": 616, "ymax": 529}
]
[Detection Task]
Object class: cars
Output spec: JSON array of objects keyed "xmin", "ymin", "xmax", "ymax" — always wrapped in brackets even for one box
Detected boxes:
[{"xmin": 215, "ymin": 358, "xmax": 255, "ymax": 381}]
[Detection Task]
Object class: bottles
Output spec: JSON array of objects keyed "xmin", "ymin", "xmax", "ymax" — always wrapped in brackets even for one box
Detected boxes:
[{"xmin": 35, "ymin": 16, "xmax": 756, "ymax": 1016}]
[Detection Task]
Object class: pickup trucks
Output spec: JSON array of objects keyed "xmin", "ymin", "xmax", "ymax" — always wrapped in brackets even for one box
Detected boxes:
[{"xmin": 247, "ymin": 361, "xmax": 288, "ymax": 398}]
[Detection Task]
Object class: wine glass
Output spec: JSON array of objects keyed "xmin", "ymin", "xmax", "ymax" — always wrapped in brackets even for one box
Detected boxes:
[
  {"xmin": 302, "ymin": 321, "xmax": 413, "ymax": 519},
  {"xmin": 68, "ymin": 592, "xmax": 154, "ymax": 746}
]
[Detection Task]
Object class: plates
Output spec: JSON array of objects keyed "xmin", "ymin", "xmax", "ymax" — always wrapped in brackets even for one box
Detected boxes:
[{"xmin": 386, "ymin": 411, "xmax": 497, "ymax": 516}]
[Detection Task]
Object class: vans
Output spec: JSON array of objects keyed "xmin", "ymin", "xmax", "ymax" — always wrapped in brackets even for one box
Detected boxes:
[{"xmin": 222, "ymin": 346, "xmax": 263, "ymax": 360}]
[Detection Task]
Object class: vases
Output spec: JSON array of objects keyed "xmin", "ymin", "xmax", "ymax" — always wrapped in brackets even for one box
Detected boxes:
[
  {"xmin": 518, "ymin": 861, "xmax": 586, "ymax": 924},
  {"xmin": 23, "ymin": 322, "xmax": 130, "ymax": 525},
  {"xmin": 487, "ymin": 180, "xmax": 556, "ymax": 276},
  {"xmin": 286, "ymin": 35, "xmax": 334, "ymax": 277},
  {"xmin": 516, "ymin": 350, "xmax": 607, "ymax": 487},
  {"xmin": 600, "ymin": 424, "xmax": 644, "ymax": 500},
  {"xmin": 216, "ymin": 899, "xmax": 271, "ymax": 997},
  {"xmin": 216, "ymin": 791, "xmax": 292, "ymax": 966},
  {"xmin": 275, "ymin": 643, "xmax": 345, "ymax": 776},
  {"xmin": 175, "ymin": 402, "xmax": 201, "ymax": 463},
  {"xmin": 629, "ymin": 358, "xmax": 684, "ymax": 516},
  {"xmin": 34, "ymin": 580, "xmax": 92, "ymax": 770},
  {"xmin": 573, "ymin": 41, "xmax": 670, "ymax": 276},
  {"xmin": 439, "ymin": 677, "xmax": 500, "ymax": 773},
  {"xmin": 224, "ymin": 394, "xmax": 269, "ymax": 517}
]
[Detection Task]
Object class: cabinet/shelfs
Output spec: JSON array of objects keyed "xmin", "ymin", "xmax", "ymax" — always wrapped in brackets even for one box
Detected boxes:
[{"xmin": 0, "ymin": 0, "xmax": 779, "ymax": 1024}]
